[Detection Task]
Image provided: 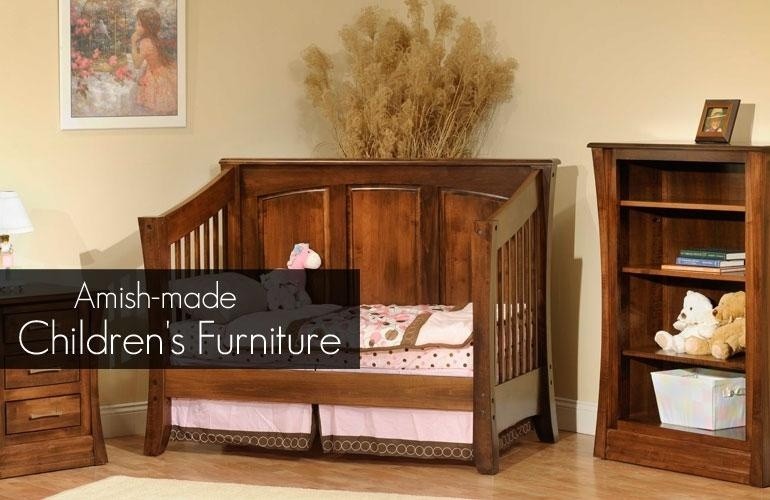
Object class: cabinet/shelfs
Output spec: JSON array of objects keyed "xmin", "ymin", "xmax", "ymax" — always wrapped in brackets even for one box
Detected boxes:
[{"xmin": 586, "ymin": 141, "xmax": 770, "ymax": 489}]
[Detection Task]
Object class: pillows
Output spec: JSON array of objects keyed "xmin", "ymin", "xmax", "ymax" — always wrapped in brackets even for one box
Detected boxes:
[{"xmin": 166, "ymin": 272, "xmax": 271, "ymax": 325}]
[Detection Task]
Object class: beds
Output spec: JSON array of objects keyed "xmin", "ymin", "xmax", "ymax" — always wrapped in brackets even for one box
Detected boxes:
[{"xmin": 137, "ymin": 157, "xmax": 563, "ymax": 475}]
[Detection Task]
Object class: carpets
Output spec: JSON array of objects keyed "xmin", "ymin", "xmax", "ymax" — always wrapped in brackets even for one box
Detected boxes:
[{"xmin": 46, "ymin": 474, "xmax": 469, "ymax": 500}]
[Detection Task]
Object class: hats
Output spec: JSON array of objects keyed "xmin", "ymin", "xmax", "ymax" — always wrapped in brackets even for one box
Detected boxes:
[{"xmin": 708, "ymin": 108, "xmax": 726, "ymax": 119}]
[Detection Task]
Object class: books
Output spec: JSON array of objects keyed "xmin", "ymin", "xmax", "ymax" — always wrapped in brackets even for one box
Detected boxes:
[{"xmin": 660, "ymin": 247, "xmax": 746, "ymax": 273}]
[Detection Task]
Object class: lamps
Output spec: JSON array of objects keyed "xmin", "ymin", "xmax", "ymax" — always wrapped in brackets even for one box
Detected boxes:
[{"xmin": 0, "ymin": 191, "xmax": 34, "ymax": 293}]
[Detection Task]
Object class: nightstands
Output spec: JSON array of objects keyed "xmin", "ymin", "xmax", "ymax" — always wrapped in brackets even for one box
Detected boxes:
[{"xmin": 0, "ymin": 282, "xmax": 109, "ymax": 480}]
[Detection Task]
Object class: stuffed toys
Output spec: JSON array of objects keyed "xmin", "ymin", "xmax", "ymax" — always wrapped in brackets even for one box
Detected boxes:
[
  {"xmin": 683, "ymin": 290, "xmax": 746, "ymax": 360},
  {"xmin": 261, "ymin": 243, "xmax": 322, "ymax": 312},
  {"xmin": 655, "ymin": 291, "xmax": 718, "ymax": 354}
]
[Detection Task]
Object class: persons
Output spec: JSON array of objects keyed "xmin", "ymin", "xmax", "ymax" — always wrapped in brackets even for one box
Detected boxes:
[
  {"xmin": 130, "ymin": 6, "xmax": 177, "ymax": 115},
  {"xmin": 705, "ymin": 107, "xmax": 722, "ymax": 133}
]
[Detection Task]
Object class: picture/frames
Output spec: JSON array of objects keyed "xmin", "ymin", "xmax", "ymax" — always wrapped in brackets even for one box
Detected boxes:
[
  {"xmin": 57, "ymin": 0, "xmax": 188, "ymax": 130},
  {"xmin": 695, "ymin": 97, "xmax": 741, "ymax": 145}
]
